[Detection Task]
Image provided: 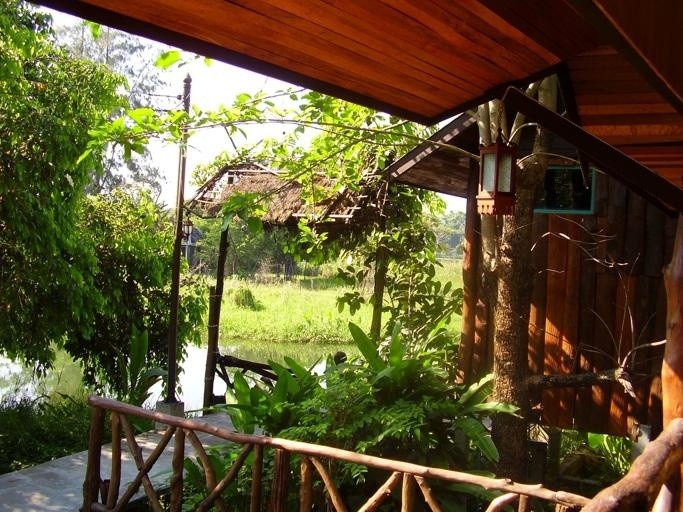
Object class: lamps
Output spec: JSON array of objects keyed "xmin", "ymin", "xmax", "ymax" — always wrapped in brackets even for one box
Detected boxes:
[{"xmin": 474, "ymin": 103, "xmax": 517, "ymax": 218}]
